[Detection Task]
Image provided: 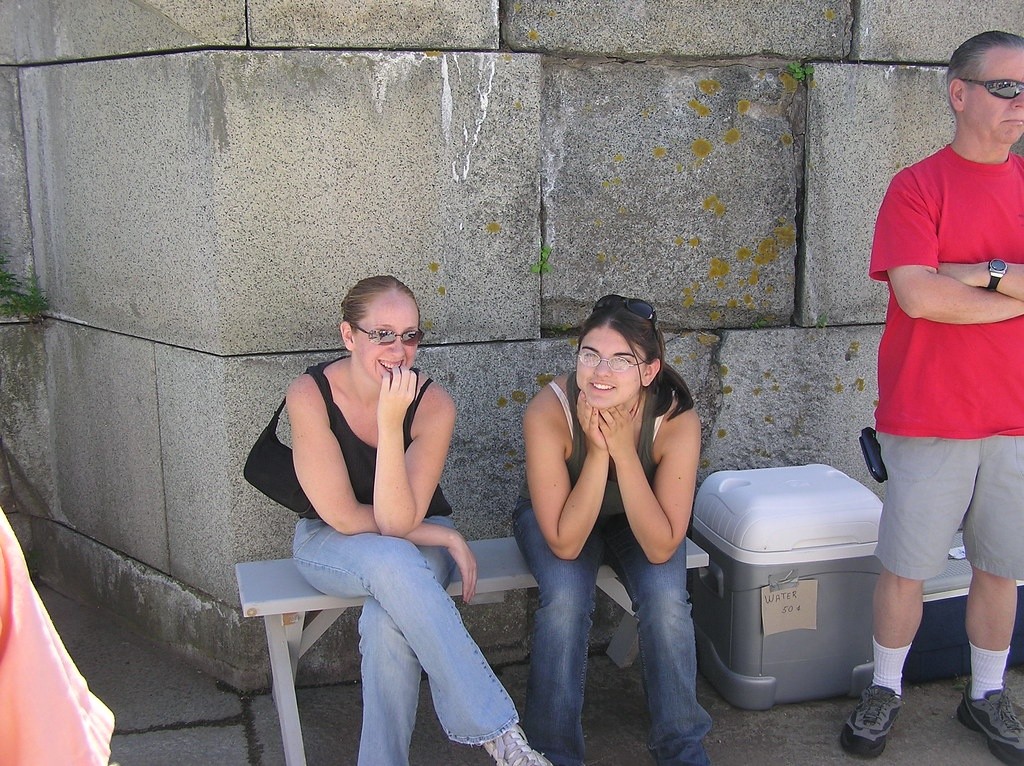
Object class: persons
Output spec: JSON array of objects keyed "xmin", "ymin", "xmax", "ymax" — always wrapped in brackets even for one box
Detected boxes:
[
  {"xmin": 0, "ymin": 505, "xmax": 116, "ymax": 766},
  {"xmin": 840, "ymin": 30, "xmax": 1024, "ymax": 766},
  {"xmin": 511, "ymin": 293, "xmax": 714, "ymax": 766},
  {"xmin": 285, "ymin": 276, "xmax": 553, "ymax": 766}
]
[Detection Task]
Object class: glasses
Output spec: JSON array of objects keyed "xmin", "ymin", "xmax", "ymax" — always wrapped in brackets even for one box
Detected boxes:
[
  {"xmin": 350, "ymin": 322, "xmax": 425, "ymax": 346},
  {"xmin": 595, "ymin": 294, "xmax": 662, "ymax": 351},
  {"xmin": 953, "ymin": 76, "xmax": 1024, "ymax": 99}
]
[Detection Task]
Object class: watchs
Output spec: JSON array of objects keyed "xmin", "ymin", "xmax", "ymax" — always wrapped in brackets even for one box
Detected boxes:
[{"xmin": 986, "ymin": 259, "xmax": 1008, "ymax": 292}]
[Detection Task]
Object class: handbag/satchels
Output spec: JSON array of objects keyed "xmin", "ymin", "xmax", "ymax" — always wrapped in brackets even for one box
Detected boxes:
[{"xmin": 243, "ymin": 369, "xmax": 334, "ymax": 515}]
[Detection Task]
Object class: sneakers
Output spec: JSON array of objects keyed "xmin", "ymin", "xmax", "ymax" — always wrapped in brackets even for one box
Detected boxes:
[
  {"xmin": 483, "ymin": 724, "xmax": 553, "ymax": 766},
  {"xmin": 839, "ymin": 679, "xmax": 903, "ymax": 758},
  {"xmin": 956, "ymin": 675, "xmax": 1024, "ymax": 765}
]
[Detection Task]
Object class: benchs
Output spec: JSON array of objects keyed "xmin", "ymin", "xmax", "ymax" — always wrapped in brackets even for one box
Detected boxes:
[{"xmin": 234, "ymin": 537, "xmax": 708, "ymax": 766}]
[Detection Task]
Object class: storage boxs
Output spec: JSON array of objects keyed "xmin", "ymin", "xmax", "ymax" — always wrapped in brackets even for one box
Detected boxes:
[{"xmin": 691, "ymin": 464, "xmax": 1023, "ymax": 710}]
